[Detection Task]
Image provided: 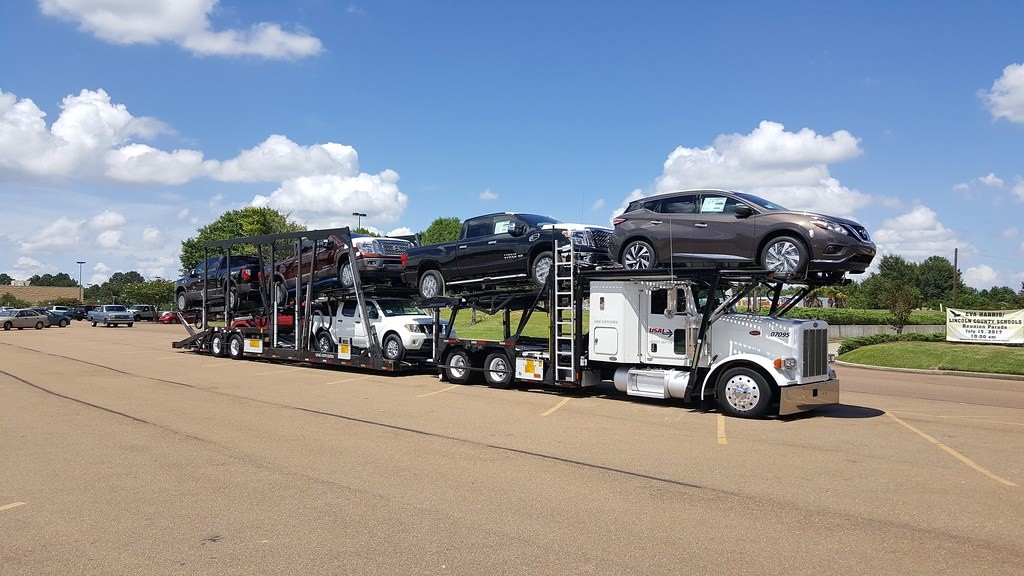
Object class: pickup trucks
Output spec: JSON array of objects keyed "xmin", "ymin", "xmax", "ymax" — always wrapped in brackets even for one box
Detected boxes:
[
  {"xmin": 230, "ymin": 298, "xmax": 313, "ymax": 334},
  {"xmin": 87, "ymin": 305, "xmax": 135, "ymax": 327},
  {"xmin": 310, "ymin": 297, "xmax": 457, "ymax": 361},
  {"xmin": 257, "ymin": 233, "xmax": 414, "ymax": 303},
  {"xmin": 128, "ymin": 304, "xmax": 165, "ymax": 322},
  {"xmin": 173, "ymin": 254, "xmax": 270, "ymax": 313},
  {"xmin": 400, "ymin": 211, "xmax": 614, "ymax": 301},
  {"xmin": 46, "ymin": 302, "xmax": 101, "ymax": 321}
]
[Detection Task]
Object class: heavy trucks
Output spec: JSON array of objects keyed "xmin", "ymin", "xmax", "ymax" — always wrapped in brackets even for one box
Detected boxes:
[{"xmin": 169, "ymin": 226, "xmax": 840, "ymax": 420}]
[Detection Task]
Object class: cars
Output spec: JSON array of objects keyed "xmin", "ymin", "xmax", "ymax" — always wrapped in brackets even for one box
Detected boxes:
[
  {"xmin": 32, "ymin": 308, "xmax": 70, "ymax": 328},
  {"xmin": 159, "ymin": 308, "xmax": 226, "ymax": 325},
  {"xmin": 0, "ymin": 308, "xmax": 49, "ymax": 331}
]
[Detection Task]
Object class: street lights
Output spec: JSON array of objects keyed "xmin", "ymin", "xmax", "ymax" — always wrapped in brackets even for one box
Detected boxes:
[
  {"xmin": 77, "ymin": 261, "xmax": 87, "ymax": 301},
  {"xmin": 351, "ymin": 212, "xmax": 366, "ymax": 230}
]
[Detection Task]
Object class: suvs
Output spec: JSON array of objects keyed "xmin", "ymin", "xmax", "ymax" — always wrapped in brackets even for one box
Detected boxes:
[{"xmin": 606, "ymin": 189, "xmax": 877, "ymax": 285}]
